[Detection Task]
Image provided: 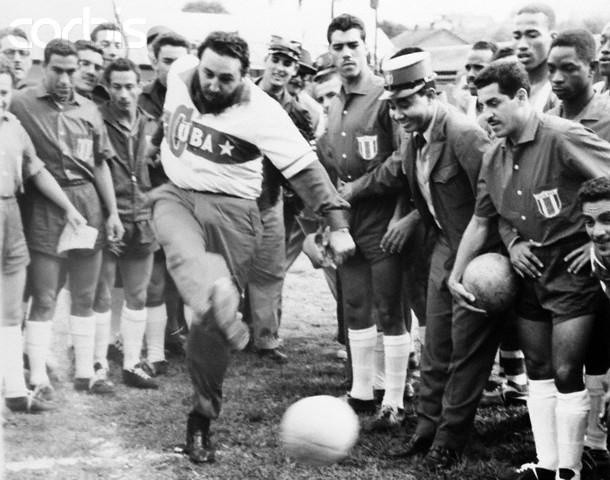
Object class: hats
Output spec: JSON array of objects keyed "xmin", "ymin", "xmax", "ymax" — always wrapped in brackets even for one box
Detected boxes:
[
  {"xmin": 267, "ymin": 34, "xmax": 308, "ymax": 64},
  {"xmin": 377, "ymin": 52, "xmax": 439, "ymax": 104},
  {"xmin": 311, "ymin": 54, "xmax": 338, "ymax": 83},
  {"xmin": 296, "ymin": 50, "xmax": 325, "ymax": 78},
  {"xmin": 144, "ymin": 25, "xmax": 178, "ymax": 44}
]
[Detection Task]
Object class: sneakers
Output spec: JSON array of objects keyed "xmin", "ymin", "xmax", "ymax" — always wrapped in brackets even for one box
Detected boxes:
[
  {"xmin": 72, "ymin": 374, "xmax": 116, "ymax": 395},
  {"xmin": 32, "ymin": 382, "xmax": 59, "ymax": 405},
  {"xmin": 122, "ymin": 367, "xmax": 161, "ymax": 389},
  {"xmin": 5, "ymin": 395, "xmax": 49, "ymax": 416},
  {"xmin": 376, "ymin": 407, "xmax": 405, "ymax": 434},
  {"xmin": 338, "ymin": 392, "xmax": 380, "ymax": 418},
  {"xmin": 136, "ymin": 361, "xmax": 178, "ymax": 378},
  {"xmin": 581, "ymin": 446, "xmax": 609, "ymax": 480},
  {"xmin": 510, "ymin": 463, "xmax": 584, "ymax": 480},
  {"xmin": 481, "ymin": 376, "xmax": 531, "ymax": 407}
]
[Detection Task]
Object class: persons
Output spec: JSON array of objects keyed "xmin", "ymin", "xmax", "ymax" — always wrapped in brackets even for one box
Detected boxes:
[
  {"xmin": 303, "ymin": 14, "xmax": 412, "ymax": 435},
  {"xmin": 334, "ymin": 51, "xmax": 544, "ymax": 467},
  {"xmin": 511, "ymin": 1, "xmax": 560, "ymax": 114},
  {"xmin": 1, "ymin": 26, "xmax": 33, "ymax": 90},
  {"xmin": 378, "ymin": 46, "xmax": 477, "ymax": 349},
  {"xmin": 137, "ymin": 30, "xmax": 194, "ymax": 357},
  {"xmin": 88, "ymin": 56, "xmax": 161, "ymax": 396},
  {"xmin": 313, "ymin": 68, "xmax": 349, "ymax": 117},
  {"xmin": 462, "ymin": 41, "xmax": 529, "ymax": 406},
  {"xmin": 247, "ymin": 35, "xmax": 316, "ymax": 365},
  {"xmin": 446, "ymin": 56, "xmax": 610, "ymax": 476},
  {"xmin": 89, "ymin": 23, "xmax": 127, "ymax": 71},
  {"xmin": 155, "ymin": 32, "xmax": 357, "ymax": 464},
  {"xmin": 144, "ymin": 25, "xmax": 172, "ymax": 71},
  {"xmin": 11, "ymin": 38, "xmax": 123, "ymax": 400},
  {"xmin": 286, "ymin": 46, "xmax": 326, "ymax": 265},
  {"xmin": 592, "ymin": 23, "xmax": 610, "ymax": 107},
  {"xmin": 576, "ymin": 177, "xmax": 610, "ymax": 285},
  {"xmin": 75, "ymin": 39, "xmax": 106, "ymax": 100},
  {"xmin": 0, "ymin": 67, "xmax": 86, "ymax": 413},
  {"xmin": 540, "ymin": 29, "xmax": 610, "ymax": 476}
]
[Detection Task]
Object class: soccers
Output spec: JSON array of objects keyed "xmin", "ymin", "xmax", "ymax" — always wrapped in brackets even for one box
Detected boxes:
[
  {"xmin": 278, "ymin": 394, "xmax": 362, "ymax": 465},
  {"xmin": 462, "ymin": 251, "xmax": 516, "ymax": 313}
]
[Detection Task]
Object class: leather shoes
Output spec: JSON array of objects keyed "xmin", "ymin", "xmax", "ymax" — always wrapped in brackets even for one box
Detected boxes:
[
  {"xmin": 185, "ymin": 417, "xmax": 214, "ymax": 464},
  {"xmin": 208, "ymin": 279, "xmax": 252, "ymax": 353},
  {"xmin": 265, "ymin": 347, "xmax": 291, "ymax": 364},
  {"xmin": 169, "ymin": 333, "xmax": 193, "ymax": 361},
  {"xmin": 387, "ymin": 433, "xmax": 426, "ymax": 459},
  {"xmin": 412, "ymin": 450, "xmax": 457, "ymax": 474}
]
